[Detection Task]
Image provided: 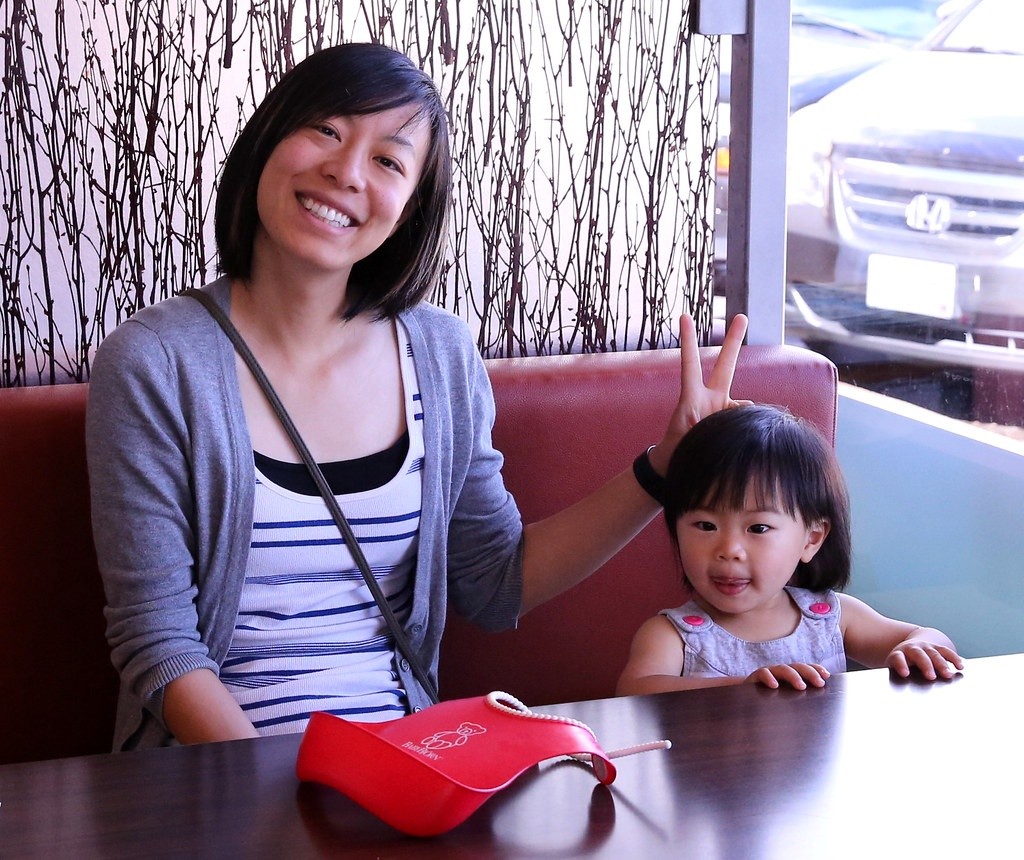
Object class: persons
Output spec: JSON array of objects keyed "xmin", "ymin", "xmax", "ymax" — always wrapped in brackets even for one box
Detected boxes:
[
  {"xmin": 84, "ymin": 42, "xmax": 753, "ymax": 755},
  {"xmin": 616, "ymin": 405, "xmax": 965, "ymax": 697}
]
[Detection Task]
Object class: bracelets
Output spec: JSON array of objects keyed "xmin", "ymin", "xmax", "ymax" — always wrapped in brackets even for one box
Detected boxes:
[{"xmin": 632, "ymin": 445, "xmax": 664, "ymax": 504}]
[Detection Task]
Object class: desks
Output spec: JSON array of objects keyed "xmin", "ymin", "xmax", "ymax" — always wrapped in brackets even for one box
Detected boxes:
[{"xmin": 0, "ymin": 651, "xmax": 1024, "ymax": 860}]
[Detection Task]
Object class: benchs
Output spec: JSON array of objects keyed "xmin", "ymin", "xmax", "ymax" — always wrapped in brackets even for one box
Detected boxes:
[{"xmin": 0, "ymin": 344, "xmax": 837, "ymax": 765}]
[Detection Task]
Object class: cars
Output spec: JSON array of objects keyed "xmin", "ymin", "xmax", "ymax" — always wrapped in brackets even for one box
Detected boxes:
[{"xmin": 712, "ymin": 1, "xmax": 1024, "ymax": 432}]
